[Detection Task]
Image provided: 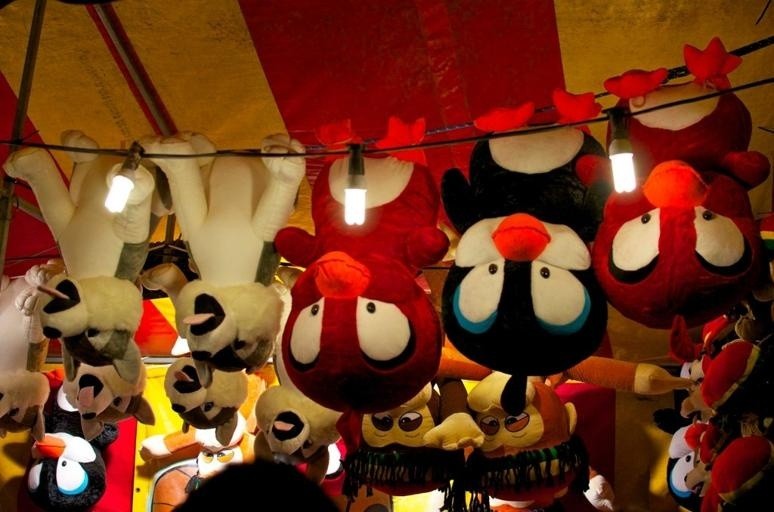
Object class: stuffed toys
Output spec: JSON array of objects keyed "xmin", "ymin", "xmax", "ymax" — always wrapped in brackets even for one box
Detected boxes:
[
  {"xmin": 337, "ymin": 371, "xmax": 487, "ymax": 498},
  {"xmin": 24, "ymin": 367, "xmax": 119, "ymax": 511},
  {"xmin": 434, "ymin": 345, "xmax": 695, "ymax": 506},
  {"xmin": 248, "ymin": 378, "xmax": 349, "ymax": 484},
  {"xmin": 158, "ymin": 349, "xmax": 253, "ymax": 446},
  {"xmin": 1, "ymin": 264, "xmax": 53, "ymax": 445},
  {"xmin": 485, "ymin": 460, "xmax": 618, "ymax": 511},
  {"xmin": 272, "ymin": 117, "xmax": 452, "ymax": 415},
  {"xmin": 128, "ymin": 133, "xmax": 312, "ymax": 447},
  {"xmin": 1, "ymin": 128, "xmax": 209, "ymax": 448},
  {"xmin": 587, "ymin": 32, "xmax": 771, "ymax": 333},
  {"xmin": 645, "ymin": 211, "xmax": 773, "ymax": 512},
  {"xmin": 433, "ymin": 83, "xmax": 618, "ymax": 420},
  {"xmin": 140, "ymin": 411, "xmax": 255, "ymax": 482}
]
